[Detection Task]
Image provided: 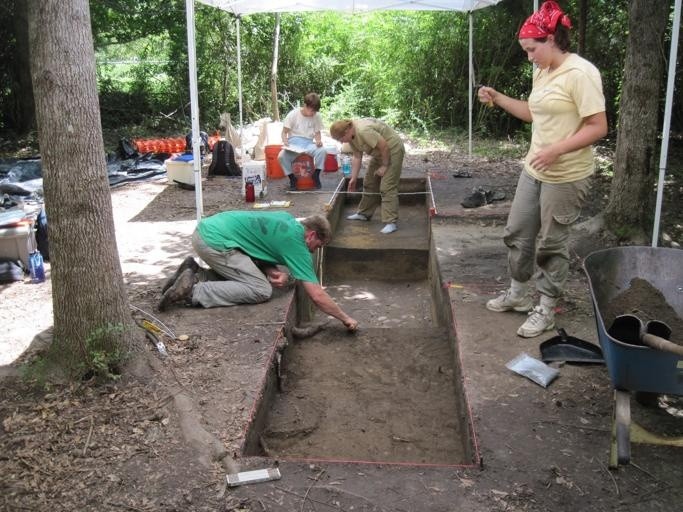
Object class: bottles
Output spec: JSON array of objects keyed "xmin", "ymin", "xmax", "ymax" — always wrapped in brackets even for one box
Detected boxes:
[
  {"xmin": 343, "ymin": 156, "xmax": 351, "ymax": 174},
  {"xmin": 245, "ymin": 177, "xmax": 255, "ymax": 202},
  {"xmin": 28, "ymin": 249, "xmax": 45, "ymax": 283}
]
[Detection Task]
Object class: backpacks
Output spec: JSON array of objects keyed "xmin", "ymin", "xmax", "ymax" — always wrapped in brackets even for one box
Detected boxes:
[
  {"xmin": 208, "ymin": 140, "xmax": 241, "ymax": 176},
  {"xmin": 120, "ymin": 136, "xmax": 139, "ymax": 159}
]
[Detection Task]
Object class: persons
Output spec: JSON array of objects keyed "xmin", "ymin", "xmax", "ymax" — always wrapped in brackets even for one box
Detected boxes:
[
  {"xmin": 157, "ymin": 210, "xmax": 359, "ymax": 333},
  {"xmin": 276, "ymin": 94, "xmax": 327, "ymax": 191},
  {"xmin": 478, "ymin": 1, "xmax": 608, "ymax": 339},
  {"xmin": 330, "ymin": 117, "xmax": 405, "ymax": 234}
]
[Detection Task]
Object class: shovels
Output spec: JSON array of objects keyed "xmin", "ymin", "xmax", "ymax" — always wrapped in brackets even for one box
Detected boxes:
[{"xmin": 606, "ymin": 315, "xmax": 683, "ymax": 358}]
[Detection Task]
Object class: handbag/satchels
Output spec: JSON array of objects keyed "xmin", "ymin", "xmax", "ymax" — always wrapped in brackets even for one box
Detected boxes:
[{"xmin": 37, "ymin": 208, "xmax": 49, "ymax": 261}]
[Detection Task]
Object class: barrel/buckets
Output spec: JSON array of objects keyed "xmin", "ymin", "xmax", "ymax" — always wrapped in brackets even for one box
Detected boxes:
[
  {"xmin": 325, "ymin": 152, "xmax": 339, "ymax": 172},
  {"xmin": 291, "ymin": 155, "xmax": 317, "ymax": 190},
  {"xmin": 265, "ymin": 145, "xmax": 286, "ymax": 178}
]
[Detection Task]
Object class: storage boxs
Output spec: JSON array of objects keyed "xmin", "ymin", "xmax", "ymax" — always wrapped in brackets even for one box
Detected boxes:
[
  {"xmin": 0, "ymin": 209, "xmax": 36, "ymax": 268},
  {"xmin": 165, "ymin": 159, "xmax": 194, "ymax": 185}
]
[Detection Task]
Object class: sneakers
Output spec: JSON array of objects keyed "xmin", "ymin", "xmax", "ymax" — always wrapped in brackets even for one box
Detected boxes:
[
  {"xmin": 478, "ymin": 185, "xmax": 505, "ymax": 205},
  {"xmin": 485, "ymin": 288, "xmax": 534, "ymax": 313},
  {"xmin": 453, "ymin": 169, "xmax": 471, "ymax": 178},
  {"xmin": 517, "ymin": 306, "xmax": 555, "ymax": 338},
  {"xmin": 163, "ymin": 255, "xmax": 199, "ymax": 295},
  {"xmin": 156, "ymin": 269, "xmax": 199, "ymax": 310},
  {"xmin": 461, "ymin": 191, "xmax": 487, "ymax": 208}
]
